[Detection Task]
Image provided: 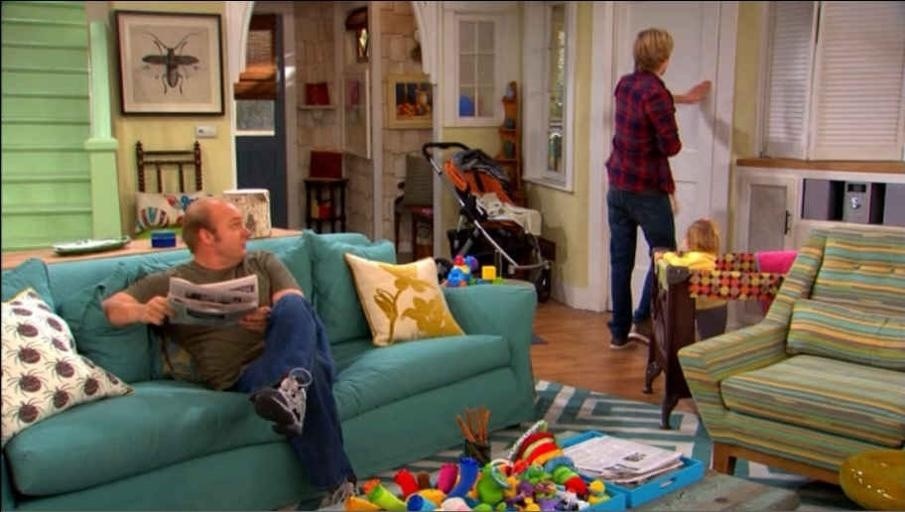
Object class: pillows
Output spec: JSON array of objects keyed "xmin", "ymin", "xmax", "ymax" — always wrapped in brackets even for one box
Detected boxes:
[
  {"xmin": 345, "ymin": 253, "xmax": 468, "ymax": 347},
  {"xmin": 1, "ymin": 255, "xmax": 60, "ymax": 325},
  {"xmin": 1, "ymin": 285, "xmax": 136, "ymax": 452},
  {"xmin": 58, "ymin": 264, "xmax": 154, "ymax": 384},
  {"xmin": 137, "ymin": 242, "xmax": 312, "ymax": 381},
  {"xmin": 398, "ymin": 150, "xmax": 435, "ymax": 209},
  {"xmin": 785, "ymin": 295, "xmax": 905, "ymax": 373},
  {"xmin": 304, "ymin": 233, "xmax": 395, "ymax": 346},
  {"xmin": 128, "ymin": 187, "xmax": 214, "ymax": 234}
]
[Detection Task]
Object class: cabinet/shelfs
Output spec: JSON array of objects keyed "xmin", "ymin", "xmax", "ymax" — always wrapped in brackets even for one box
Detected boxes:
[{"xmin": 726, "ymin": 162, "xmax": 801, "ymax": 255}]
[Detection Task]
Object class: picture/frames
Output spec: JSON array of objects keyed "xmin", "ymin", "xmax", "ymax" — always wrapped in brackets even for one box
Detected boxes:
[
  {"xmin": 384, "ymin": 71, "xmax": 434, "ymax": 131},
  {"xmin": 112, "ymin": 5, "xmax": 228, "ymax": 118}
]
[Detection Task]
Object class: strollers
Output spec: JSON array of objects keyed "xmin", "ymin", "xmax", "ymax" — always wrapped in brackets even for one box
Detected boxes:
[{"xmin": 413, "ymin": 139, "xmax": 559, "ymax": 305}]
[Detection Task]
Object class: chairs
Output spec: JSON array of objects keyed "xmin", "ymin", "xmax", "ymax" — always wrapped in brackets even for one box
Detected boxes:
[
  {"xmin": 130, "ymin": 139, "xmax": 206, "ymax": 238},
  {"xmin": 668, "ymin": 215, "xmax": 905, "ymax": 496},
  {"xmin": 387, "ymin": 181, "xmax": 434, "ymax": 263}
]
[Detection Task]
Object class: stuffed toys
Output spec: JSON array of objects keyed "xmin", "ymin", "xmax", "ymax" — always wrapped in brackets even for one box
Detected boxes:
[
  {"xmin": 343, "ymin": 420, "xmax": 609, "ymax": 512},
  {"xmin": 445, "ymin": 255, "xmax": 508, "ymax": 288}
]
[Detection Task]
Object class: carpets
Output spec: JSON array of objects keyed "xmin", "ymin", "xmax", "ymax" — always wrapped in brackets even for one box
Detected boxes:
[{"xmin": 276, "ymin": 372, "xmax": 841, "ymax": 510}]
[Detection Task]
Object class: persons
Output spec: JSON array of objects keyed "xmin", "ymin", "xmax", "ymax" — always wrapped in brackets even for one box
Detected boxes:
[
  {"xmin": 651, "ymin": 219, "xmax": 729, "ymax": 345},
  {"xmin": 602, "ymin": 25, "xmax": 715, "ymax": 349},
  {"xmin": 100, "ymin": 198, "xmax": 358, "ymax": 507}
]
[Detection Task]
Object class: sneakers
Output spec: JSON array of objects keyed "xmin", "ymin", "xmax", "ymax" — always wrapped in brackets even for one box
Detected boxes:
[
  {"xmin": 628, "ymin": 320, "xmax": 654, "ymax": 345},
  {"xmin": 252, "ymin": 377, "xmax": 310, "ymax": 434},
  {"xmin": 318, "ymin": 481, "xmax": 360, "ymax": 507},
  {"xmin": 612, "ymin": 337, "xmax": 633, "ymax": 351}
]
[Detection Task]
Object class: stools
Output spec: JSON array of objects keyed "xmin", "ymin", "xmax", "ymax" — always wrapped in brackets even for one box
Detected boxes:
[{"xmin": 301, "ymin": 174, "xmax": 350, "ymax": 235}]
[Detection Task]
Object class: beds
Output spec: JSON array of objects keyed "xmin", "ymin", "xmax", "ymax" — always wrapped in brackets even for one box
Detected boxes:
[{"xmin": 639, "ymin": 238, "xmax": 801, "ymax": 432}]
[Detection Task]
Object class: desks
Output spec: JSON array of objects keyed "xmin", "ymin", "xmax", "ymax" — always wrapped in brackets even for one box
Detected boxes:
[{"xmin": 2, "ymin": 224, "xmax": 306, "ymax": 272}]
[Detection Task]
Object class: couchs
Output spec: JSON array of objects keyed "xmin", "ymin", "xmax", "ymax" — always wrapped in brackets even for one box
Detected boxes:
[{"xmin": 2, "ymin": 221, "xmax": 546, "ymax": 512}]
